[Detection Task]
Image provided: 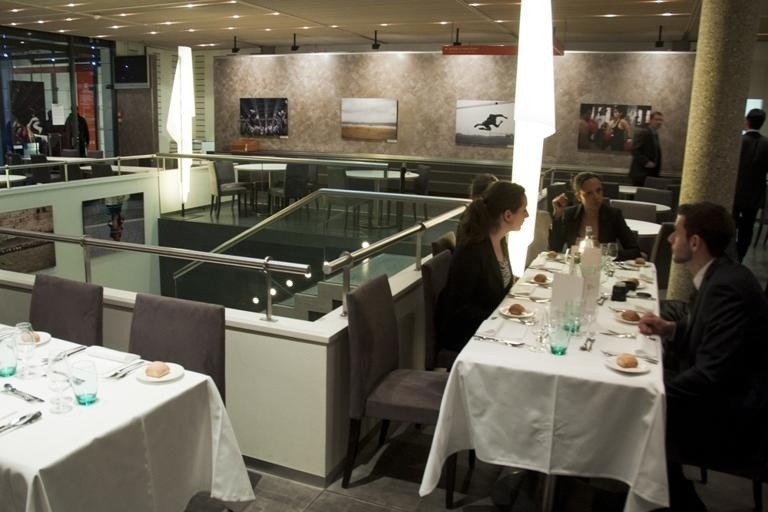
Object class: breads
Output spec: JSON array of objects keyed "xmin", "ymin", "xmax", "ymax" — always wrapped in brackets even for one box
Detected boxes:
[
  {"xmin": 21, "ymin": 333, "xmax": 40, "ymax": 342},
  {"xmin": 145, "ymin": 360, "xmax": 169, "ymax": 377},
  {"xmin": 621, "ymin": 310, "xmax": 641, "ymax": 321},
  {"xmin": 509, "ymin": 303, "xmax": 525, "ymax": 314},
  {"xmin": 617, "ymin": 354, "xmax": 638, "ymax": 368},
  {"xmin": 534, "ymin": 273, "xmax": 547, "ymax": 283}
]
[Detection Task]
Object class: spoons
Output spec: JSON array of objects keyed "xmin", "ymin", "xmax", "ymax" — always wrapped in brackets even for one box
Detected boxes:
[{"xmin": 473, "ymin": 334, "xmax": 525, "ymax": 346}]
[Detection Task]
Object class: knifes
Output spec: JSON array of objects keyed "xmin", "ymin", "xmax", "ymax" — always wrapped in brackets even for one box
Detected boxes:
[{"xmin": 117, "ymin": 363, "xmax": 149, "ymax": 379}]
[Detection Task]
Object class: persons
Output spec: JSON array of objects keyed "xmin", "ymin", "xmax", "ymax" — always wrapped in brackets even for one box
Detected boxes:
[
  {"xmin": 733, "ymin": 108, "xmax": 768, "ymax": 263},
  {"xmin": 471, "ymin": 173, "xmax": 496, "ymax": 202},
  {"xmin": 586, "ymin": 108, "xmax": 598, "ymax": 131},
  {"xmin": 65, "ymin": 106, "xmax": 91, "ymax": 156},
  {"xmin": 629, "ymin": 112, "xmax": 664, "ymax": 184},
  {"xmin": 547, "ymin": 172, "xmax": 639, "ymax": 261},
  {"xmin": 578, "ymin": 104, "xmax": 589, "ymax": 149},
  {"xmin": 637, "ymin": 202, "xmax": 768, "ymax": 512},
  {"xmin": 434, "ymin": 181, "xmax": 530, "ymax": 370},
  {"xmin": 595, "ymin": 108, "xmax": 608, "ymax": 150},
  {"xmin": 608, "ymin": 105, "xmax": 630, "ymax": 153}
]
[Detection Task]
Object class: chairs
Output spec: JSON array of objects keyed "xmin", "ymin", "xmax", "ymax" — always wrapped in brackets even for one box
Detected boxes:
[
  {"xmin": 602, "ymin": 183, "xmax": 619, "ymax": 197},
  {"xmin": 342, "ymin": 274, "xmax": 476, "ymax": 511},
  {"xmin": 30, "ymin": 154, "xmax": 50, "ymax": 183},
  {"xmin": 127, "ymin": 292, "xmax": 227, "ymax": 407},
  {"xmin": 59, "ymin": 164, "xmax": 82, "ymax": 180},
  {"xmin": 645, "ymin": 176, "xmax": 672, "ymax": 188},
  {"xmin": 667, "ymin": 447, "xmax": 768, "ymax": 512},
  {"xmin": 215, "ymin": 154, "xmax": 247, "ymax": 219},
  {"xmin": 306, "ymin": 163, "xmax": 321, "ymax": 213},
  {"xmin": 420, "ymin": 250, "xmax": 458, "ymax": 371},
  {"xmin": 92, "ymin": 163, "xmax": 112, "ymax": 176},
  {"xmin": 386, "ymin": 168, "xmax": 428, "ymax": 226},
  {"xmin": 650, "ymin": 222, "xmax": 675, "ymax": 290},
  {"xmin": 61, "ymin": 149, "xmax": 79, "ymax": 157},
  {"xmin": 324, "ymin": 166, "xmax": 374, "ymax": 231},
  {"xmin": 29, "ymin": 275, "xmax": 104, "ymax": 347},
  {"xmin": 430, "ymin": 231, "xmax": 458, "ymax": 257},
  {"xmin": 737, "ymin": 206, "xmax": 768, "ymax": 251},
  {"xmin": 635, "ymin": 188, "xmax": 674, "ymax": 206},
  {"xmin": 611, "ymin": 201, "xmax": 657, "ymax": 222},
  {"xmin": 269, "ymin": 164, "xmax": 310, "ymax": 221},
  {"xmin": 87, "ymin": 150, "xmax": 103, "ymax": 159}
]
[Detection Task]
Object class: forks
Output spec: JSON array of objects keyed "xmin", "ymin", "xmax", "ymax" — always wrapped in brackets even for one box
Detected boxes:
[
  {"xmin": 599, "ymin": 350, "xmax": 659, "ymax": 363},
  {"xmin": 106, "ymin": 360, "xmax": 144, "ymax": 377}
]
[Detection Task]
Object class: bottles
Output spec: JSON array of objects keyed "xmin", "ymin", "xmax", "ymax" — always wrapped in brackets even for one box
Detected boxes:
[{"xmin": 584, "ymin": 225, "xmax": 594, "ymax": 248}]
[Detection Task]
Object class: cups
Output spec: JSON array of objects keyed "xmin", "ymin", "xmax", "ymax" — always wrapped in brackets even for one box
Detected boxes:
[
  {"xmin": 600, "ymin": 243, "xmax": 618, "ymax": 265},
  {"xmin": 581, "ymin": 302, "xmax": 599, "ymax": 339},
  {"xmin": 560, "ymin": 299, "xmax": 584, "ymax": 335},
  {"xmin": 69, "ymin": 360, "xmax": 99, "ymax": 406},
  {"xmin": 15, "ymin": 322, "xmax": 35, "ymax": 355},
  {"xmin": 565, "ymin": 248, "xmax": 581, "ymax": 275},
  {"xmin": 0, "ymin": 339, "xmax": 18, "ymax": 378},
  {"xmin": 548, "ymin": 312, "xmax": 572, "ymax": 356}
]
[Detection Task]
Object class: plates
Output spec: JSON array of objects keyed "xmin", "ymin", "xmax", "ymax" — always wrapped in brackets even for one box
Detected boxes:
[
  {"xmin": 499, "ymin": 305, "xmax": 534, "ymax": 318},
  {"xmin": 135, "ymin": 362, "xmax": 184, "ymax": 382},
  {"xmin": 527, "ymin": 276, "xmax": 553, "ymax": 284},
  {"xmin": 626, "ymin": 260, "xmax": 652, "ymax": 267},
  {"xmin": 611, "ymin": 312, "xmax": 645, "ymax": 325},
  {"xmin": 15, "ymin": 331, "xmax": 51, "ymax": 345},
  {"xmin": 622, "ymin": 279, "xmax": 646, "ymax": 288},
  {"xmin": 604, "ymin": 356, "xmax": 651, "ymax": 372}
]
